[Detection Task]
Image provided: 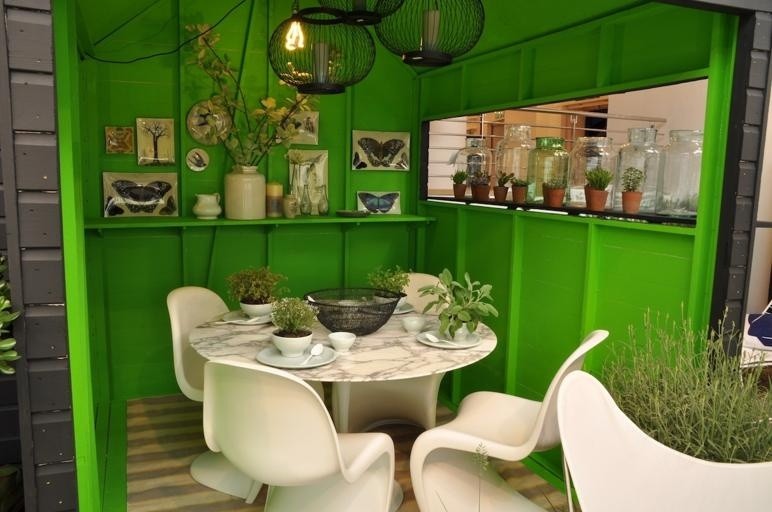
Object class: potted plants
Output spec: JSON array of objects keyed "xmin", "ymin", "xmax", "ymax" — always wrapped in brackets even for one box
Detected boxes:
[{"xmin": 450, "ymin": 168, "xmax": 647, "ymax": 215}]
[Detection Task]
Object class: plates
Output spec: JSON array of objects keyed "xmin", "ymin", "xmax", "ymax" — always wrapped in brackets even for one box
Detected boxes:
[
  {"xmin": 255, "ymin": 345, "xmax": 336, "ymax": 370},
  {"xmin": 221, "ymin": 311, "xmax": 274, "ymax": 325},
  {"xmin": 338, "ymin": 209, "xmax": 368, "ymax": 219},
  {"xmin": 359, "ymin": 302, "xmax": 417, "ymax": 317},
  {"xmin": 416, "ymin": 329, "xmax": 482, "ymax": 349}
]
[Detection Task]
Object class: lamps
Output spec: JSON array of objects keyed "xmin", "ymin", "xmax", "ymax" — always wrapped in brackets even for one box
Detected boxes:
[{"xmin": 268, "ymin": 1, "xmax": 486, "ymax": 95}]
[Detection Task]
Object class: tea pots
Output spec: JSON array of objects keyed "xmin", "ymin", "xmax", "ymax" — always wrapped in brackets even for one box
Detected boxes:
[{"xmin": 189, "ymin": 190, "xmax": 224, "ymax": 220}]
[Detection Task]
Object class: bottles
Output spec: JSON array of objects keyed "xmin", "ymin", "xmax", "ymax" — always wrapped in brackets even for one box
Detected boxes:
[
  {"xmin": 497, "ymin": 126, "xmax": 703, "ymax": 217},
  {"xmin": 453, "ymin": 132, "xmax": 493, "ymax": 192},
  {"xmin": 300, "ymin": 184, "xmax": 312, "ymax": 216},
  {"xmin": 318, "ymin": 183, "xmax": 329, "ymax": 215},
  {"xmin": 266, "ymin": 182, "xmax": 297, "ymax": 218}
]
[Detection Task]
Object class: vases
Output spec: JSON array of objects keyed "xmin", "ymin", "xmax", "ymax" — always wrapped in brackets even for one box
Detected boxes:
[{"xmin": 224, "ymin": 164, "xmax": 267, "ymax": 221}]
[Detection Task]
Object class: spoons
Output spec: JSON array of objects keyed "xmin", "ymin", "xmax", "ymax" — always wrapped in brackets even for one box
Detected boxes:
[
  {"xmin": 424, "ymin": 333, "xmax": 466, "ymax": 347},
  {"xmin": 300, "ymin": 342, "xmax": 324, "ymax": 366}
]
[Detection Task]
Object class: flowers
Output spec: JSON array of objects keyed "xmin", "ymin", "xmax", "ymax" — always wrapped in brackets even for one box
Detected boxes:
[{"xmin": 183, "ymin": 21, "xmax": 320, "ymax": 168}]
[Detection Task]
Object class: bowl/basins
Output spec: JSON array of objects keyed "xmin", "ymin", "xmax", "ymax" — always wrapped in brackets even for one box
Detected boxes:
[
  {"xmin": 303, "ymin": 286, "xmax": 401, "ymax": 337},
  {"xmin": 404, "ymin": 315, "xmax": 424, "ymax": 336},
  {"xmin": 329, "ymin": 330, "xmax": 357, "ymax": 352}
]
